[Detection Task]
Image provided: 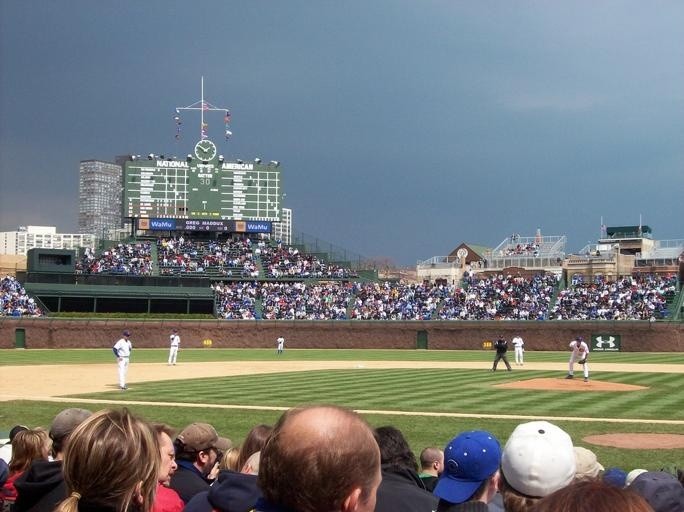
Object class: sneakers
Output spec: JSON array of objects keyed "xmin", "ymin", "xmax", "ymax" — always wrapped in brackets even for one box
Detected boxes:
[
  {"xmin": 566, "ymin": 375, "xmax": 589, "ymax": 383},
  {"xmin": 490, "ymin": 362, "xmax": 524, "ymax": 372},
  {"xmin": 121, "ymin": 384, "xmax": 128, "ymax": 390}
]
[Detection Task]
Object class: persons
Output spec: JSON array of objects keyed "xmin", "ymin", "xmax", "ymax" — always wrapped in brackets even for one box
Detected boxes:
[
  {"xmin": 490, "ymin": 334, "xmax": 511, "ymax": 371},
  {"xmin": 0, "ymin": 404, "xmax": 683, "ymax": 511},
  {"xmin": 511, "ymin": 331, "xmax": 524, "ymax": 366},
  {"xmin": 165, "ymin": 329, "xmax": 180, "ymax": 364},
  {"xmin": 563, "ymin": 335, "xmax": 589, "ymax": 382},
  {"xmin": 111, "ymin": 330, "xmax": 133, "ymax": 389},
  {"xmin": 1, "ymin": 213, "xmax": 683, "ymax": 328},
  {"xmin": 275, "ymin": 333, "xmax": 283, "ymax": 354}
]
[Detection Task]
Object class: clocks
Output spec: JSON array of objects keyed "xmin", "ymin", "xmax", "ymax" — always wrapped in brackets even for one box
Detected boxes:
[{"xmin": 195, "ymin": 139, "xmax": 217, "ymax": 162}]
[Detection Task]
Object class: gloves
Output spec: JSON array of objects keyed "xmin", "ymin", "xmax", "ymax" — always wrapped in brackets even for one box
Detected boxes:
[{"xmin": 578, "ymin": 359, "xmax": 587, "ymax": 364}]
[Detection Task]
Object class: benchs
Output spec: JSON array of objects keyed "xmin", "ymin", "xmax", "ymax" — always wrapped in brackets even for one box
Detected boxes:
[{"xmin": 654, "ymin": 286, "xmax": 683, "ymax": 319}]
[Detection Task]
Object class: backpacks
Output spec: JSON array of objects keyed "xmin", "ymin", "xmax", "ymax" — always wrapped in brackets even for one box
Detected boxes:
[{"xmin": 369, "ymin": 466, "xmax": 440, "ymax": 511}]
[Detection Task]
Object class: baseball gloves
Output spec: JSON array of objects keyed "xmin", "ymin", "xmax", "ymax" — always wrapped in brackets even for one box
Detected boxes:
[{"xmin": 578, "ymin": 359, "xmax": 586, "ymax": 364}]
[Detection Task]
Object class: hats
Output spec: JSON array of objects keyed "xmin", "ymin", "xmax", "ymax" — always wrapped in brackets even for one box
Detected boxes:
[
  {"xmin": 575, "ymin": 447, "xmax": 684, "ymax": 512},
  {"xmin": 499, "ymin": 335, "xmax": 504, "ymax": 338},
  {"xmin": 432, "ymin": 430, "xmax": 501, "ymax": 505},
  {"xmin": 175, "ymin": 423, "xmax": 232, "ymax": 453},
  {"xmin": 49, "ymin": 408, "xmax": 93, "ymax": 442},
  {"xmin": 502, "ymin": 421, "xmax": 574, "ymax": 498},
  {"xmin": 6, "ymin": 425, "xmax": 30, "ymax": 444},
  {"xmin": 575, "ymin": 336, "xmax": 583, "ymax": 341},
  {"xmin": 122, "ymin": 330, "xmax": 131, "ymax": 336}
]
